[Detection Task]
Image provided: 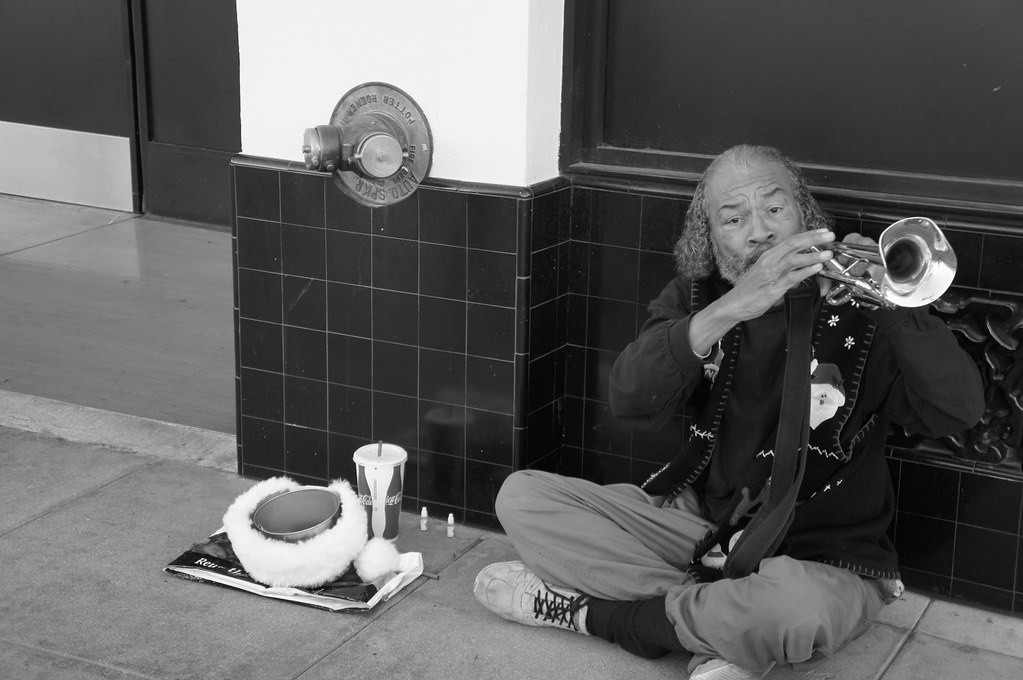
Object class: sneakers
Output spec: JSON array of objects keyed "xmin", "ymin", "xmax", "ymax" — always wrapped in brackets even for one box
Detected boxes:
[
  {"xmin": 688, "ymin": 658, "xmax": 777, "ymax": 680},
  {"xmin": 472, "ymin": 560, "xmax": 590, "ymax": 634}
]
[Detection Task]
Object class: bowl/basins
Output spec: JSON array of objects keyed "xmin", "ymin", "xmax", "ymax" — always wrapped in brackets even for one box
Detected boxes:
[{"xmin": 253, "ymin": 487, "xmax": 341, "ymax": 542}]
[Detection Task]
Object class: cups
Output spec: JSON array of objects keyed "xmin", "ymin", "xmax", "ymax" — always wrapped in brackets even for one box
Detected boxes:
[{"xmin": 352, "ymin": 443, "xmax": 409, "ymax": 542}]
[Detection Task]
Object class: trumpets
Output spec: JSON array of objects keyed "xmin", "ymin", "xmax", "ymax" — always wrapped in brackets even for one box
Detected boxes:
[{"xmin": 801, "ymin": 216, "xmax": 958, "ymax": 308}]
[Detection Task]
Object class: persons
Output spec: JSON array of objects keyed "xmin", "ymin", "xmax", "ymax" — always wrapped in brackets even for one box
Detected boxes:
[{"xmin": 474, "ymin": 143, "xmax": 986, "ymax": 680}]
